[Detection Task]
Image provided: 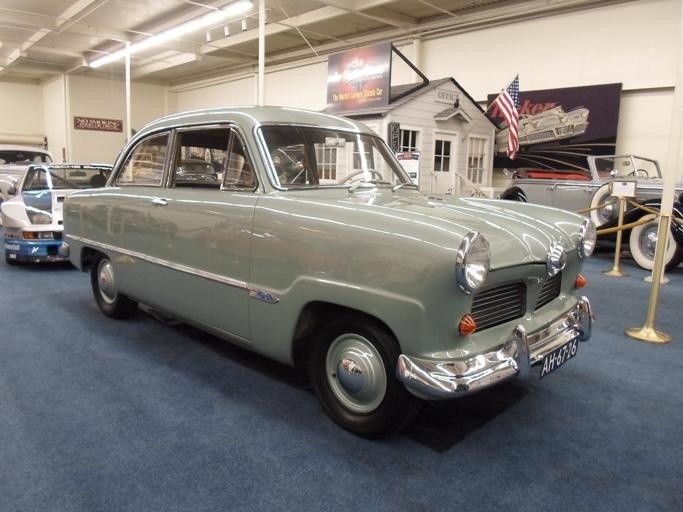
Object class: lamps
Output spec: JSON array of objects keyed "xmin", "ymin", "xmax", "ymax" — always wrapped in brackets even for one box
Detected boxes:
[{"xmin": 88, "ymin": 1, "xmax": 253, "ymax": 69}]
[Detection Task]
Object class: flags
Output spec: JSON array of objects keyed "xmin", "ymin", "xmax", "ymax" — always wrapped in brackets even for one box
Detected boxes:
[{"xmin": 495, "ymin": 74, "xmax": 520, "ymax": 160}]
